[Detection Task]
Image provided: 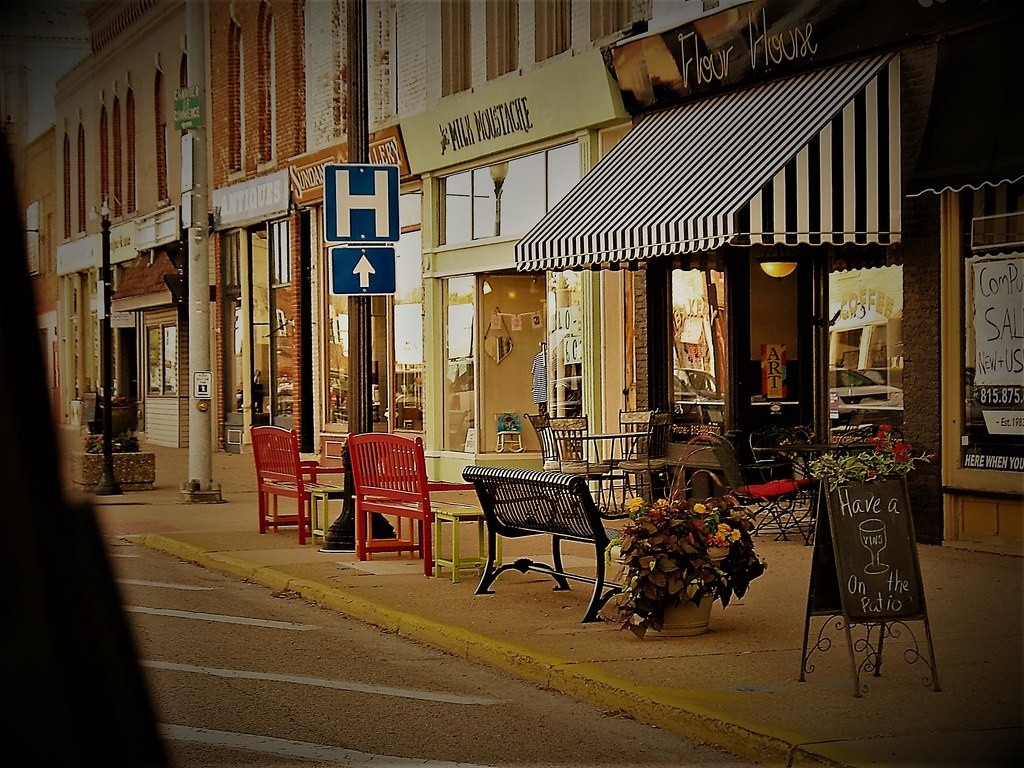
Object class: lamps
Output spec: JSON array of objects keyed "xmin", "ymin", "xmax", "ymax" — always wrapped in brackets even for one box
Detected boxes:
[
  {"xmin": 755, "ymin": 255, "xmax": 798, "ymax": 282},
  {"xmin": 482, "ymin": 280, "xmax": 493, "ymax": 295}
]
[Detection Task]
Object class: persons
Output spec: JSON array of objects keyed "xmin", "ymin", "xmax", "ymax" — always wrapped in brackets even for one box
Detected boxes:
[{"xmin": 254, "ymin": 370, "xmax": 261, "ymax": 385}]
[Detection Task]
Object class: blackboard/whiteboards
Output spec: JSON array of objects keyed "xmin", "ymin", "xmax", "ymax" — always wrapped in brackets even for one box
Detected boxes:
[
  {"xmin": 82, "ymin": 391, "xmax": 96, "ymax": 422},
  {"xmin": 823, "ymin": 473, "xmax": 929, "ymax": 624}
]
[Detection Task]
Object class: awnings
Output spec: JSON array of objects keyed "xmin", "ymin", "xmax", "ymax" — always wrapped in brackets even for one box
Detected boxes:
[{"xmin": 514, "ymin": 48, "xmax": 904, "ymax": 274}]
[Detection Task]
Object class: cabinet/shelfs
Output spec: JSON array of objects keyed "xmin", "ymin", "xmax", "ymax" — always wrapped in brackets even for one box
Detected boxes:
[{"xmin": 224, "ymin": 414, "xmax": 270, "ymax": 454}]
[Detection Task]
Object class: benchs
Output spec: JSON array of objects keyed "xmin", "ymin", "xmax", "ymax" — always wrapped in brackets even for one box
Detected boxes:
[
  {"xmin": 461, "ymin": 465, "xmax": 628, "ymax": 624},
  {"xmin": 249, "ymin": 424, "xmax": 345, "ymax": 545},
  {"xmin": 348, "ymin": 433, "xmax": 499, "ymax": 576}
]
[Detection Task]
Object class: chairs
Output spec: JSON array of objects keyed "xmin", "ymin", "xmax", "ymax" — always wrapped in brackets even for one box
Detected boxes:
[
  {"xmin": 684, "ymin": 368, "xmax": 717, "ymax": 394},
  {"xmin": 832, "ymin": 425, "xmax": 904, "ymax": 462},
  {"xmin": 492, "ymin": 410, "xmax": 524, "ymax": 453},
  {"xmin": 617, "ymin": 411, "xmax": 675, "ymax": 512},
  {"xmin": 598, "ymin": 407, "xmax": 661, "ymax": 510},
  {"xmin": 522, "ymin": 412, "xmax": 607, "ymax": 510},
  {"xmin": 545, "ymin": 413, "xmax": 611, "ymax": 516},
  {"xmin": 749, "ymin": 432, "xmax": 819, "ymax": 542},
  {"xmin": 707, "ymin": 432, "xmax": 812, "ymax": 547}
]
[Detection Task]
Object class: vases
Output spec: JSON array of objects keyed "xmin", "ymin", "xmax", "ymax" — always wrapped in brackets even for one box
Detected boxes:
[
  {"xmin": 86, "ymin": 407, "xmax": 138, "ymax": 434},
  {"xmin": 68, "ymin": 451, "xmax": 155, "ymax": 493},
  {"xmin": 636, "ymin": 587, "xmax": 712, "ymax": 637}
]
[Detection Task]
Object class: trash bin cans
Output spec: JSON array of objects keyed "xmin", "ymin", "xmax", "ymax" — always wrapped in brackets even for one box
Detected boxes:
[{"xmin": 224, "ymin": 413, "xmax": 244, "ymax": 454}]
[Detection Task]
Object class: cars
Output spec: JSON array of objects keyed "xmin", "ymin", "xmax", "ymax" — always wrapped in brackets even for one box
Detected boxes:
[{"xmin": 831, "ymin": 367, "xmax": 904, "ymax": 408}]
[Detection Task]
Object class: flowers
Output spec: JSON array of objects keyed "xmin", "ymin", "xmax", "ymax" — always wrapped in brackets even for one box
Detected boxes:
[
  {"xmin": 112, "ymin": 395, "xmax": 135, "ymax": 407},
  {"xmin": 594, "ymin": 497, "xmax": 767, "ymax": 640},
  {"xmin": 807, "ymin": 424, "xmax": 937, "ymax": 492},
  {"xmin": 81, "ymin": 433, "xmax": 142, "ymax": 454}
]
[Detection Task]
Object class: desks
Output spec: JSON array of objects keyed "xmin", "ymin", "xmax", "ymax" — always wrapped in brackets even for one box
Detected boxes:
[
  {"xmin": 556, "ymin": 432, "xmax": 653, "ymax": 515},
  {"xmin": 753, "ymin": 441, "xmax": 894, "ymax": 547}
]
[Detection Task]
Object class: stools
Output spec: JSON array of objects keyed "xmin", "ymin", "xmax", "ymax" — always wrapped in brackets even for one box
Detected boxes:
[
  {"xmin": 311, "ymin": 489, "xmax": 344, "ymax": 545},
  {"xmin": 434, "ymin": 507, "xmax": 502, "ymax": 583}
]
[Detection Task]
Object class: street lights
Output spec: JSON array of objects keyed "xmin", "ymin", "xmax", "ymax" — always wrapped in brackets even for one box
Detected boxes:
[{"xmin": 93, "ymin": 201, "xmax": 127, "ymax": 496}]
